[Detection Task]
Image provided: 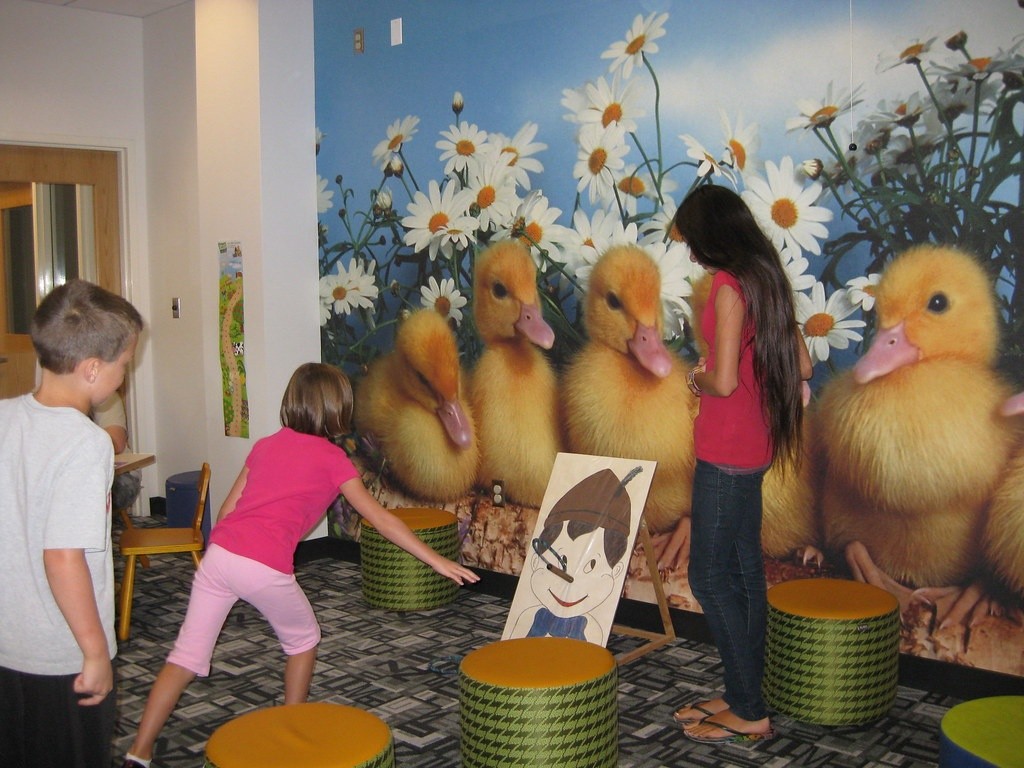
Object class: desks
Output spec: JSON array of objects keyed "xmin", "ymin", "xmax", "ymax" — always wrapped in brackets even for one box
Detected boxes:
[{"xmin": 113, "ymin": 452, "xmax": 157, "ymax": 570}]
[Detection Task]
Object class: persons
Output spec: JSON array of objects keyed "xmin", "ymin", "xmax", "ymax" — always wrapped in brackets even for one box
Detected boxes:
[
  {"xmin": 123, "ymin": 362, "xmax": 482, "ymax": 768},
  {"xmin": 86, "ymin": 389, "xmax": 144, "ymax": 521},
  {"xmin": 667, "ymin": 184, "xmax": 812, "ymax": 743},
  {"xmin": 0, "ymin": 278, "xmax": 144, "ymax": 768}
]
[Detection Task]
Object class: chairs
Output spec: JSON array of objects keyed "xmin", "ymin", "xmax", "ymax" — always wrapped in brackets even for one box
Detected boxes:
[{"xmin": 119, "ymin": 462, "xmax": 213, "ymax": 641}]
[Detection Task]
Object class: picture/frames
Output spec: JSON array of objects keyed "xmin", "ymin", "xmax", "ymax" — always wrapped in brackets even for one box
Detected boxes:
[{"xmin": 499, "ymin": 452, "xmax": 677, "ymax": 667}]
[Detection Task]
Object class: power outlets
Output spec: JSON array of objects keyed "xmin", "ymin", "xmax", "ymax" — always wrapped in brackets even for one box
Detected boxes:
[{"xmin": 171, "ymin": 297, "xmax": 181, "ymax": 319}]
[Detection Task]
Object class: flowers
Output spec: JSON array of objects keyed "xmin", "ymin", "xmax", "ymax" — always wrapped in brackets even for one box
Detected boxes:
[{"xmin": 317, "ymin": 7, "xmax": 1022, "ymax": 403}]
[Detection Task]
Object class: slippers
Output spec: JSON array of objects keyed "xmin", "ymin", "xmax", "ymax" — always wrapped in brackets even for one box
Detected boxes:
[{"xmin": 674, "ymin": 698, "xmax": 776, "ymax": 744}]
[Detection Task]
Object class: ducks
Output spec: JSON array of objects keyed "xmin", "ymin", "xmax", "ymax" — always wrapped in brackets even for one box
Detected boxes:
[{"xmin": 350, "ymin": 240, "xmax": 1023, "ymax": 633}]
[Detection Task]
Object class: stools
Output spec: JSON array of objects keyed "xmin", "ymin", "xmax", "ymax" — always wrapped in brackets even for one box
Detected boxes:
[
  {"xmin": 458, "ymin": 637, "xmax": 622, "ymax": 768},
  {"xmin": 360, "ymin": 507, "xmax": 463, "ymax": 614},
  {"xmin": 202, "ymin": 702, "xmax": 397, "ymax": 768},
  {"xmin": 939, "ymin": 694, "xmax": 1023, "ymax": 768},
  {"xmin": 765, "ymin": 577, "xmax": 901, "ymax": 728}
]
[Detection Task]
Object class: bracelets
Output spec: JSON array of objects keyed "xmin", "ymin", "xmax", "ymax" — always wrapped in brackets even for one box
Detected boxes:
[{"xmin": 687, "ymin": 368, "xmax": 704, "ymax": 392}]
